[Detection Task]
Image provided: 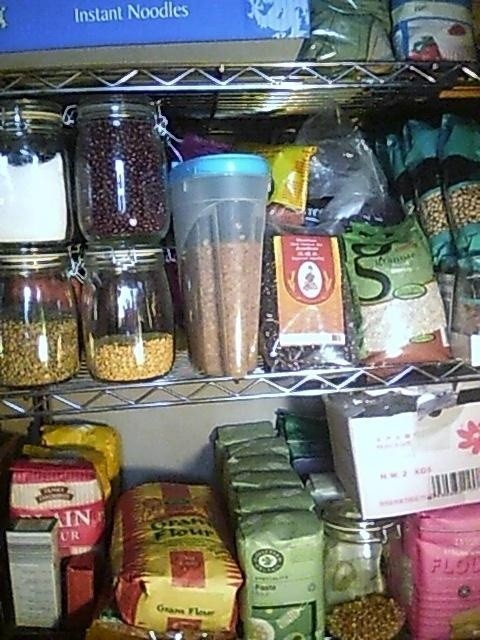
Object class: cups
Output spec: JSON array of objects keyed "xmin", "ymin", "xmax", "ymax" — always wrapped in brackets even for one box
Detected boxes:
[{"xmin": 168, "ymin": 154, "xmax": 269, "ymax": 379}]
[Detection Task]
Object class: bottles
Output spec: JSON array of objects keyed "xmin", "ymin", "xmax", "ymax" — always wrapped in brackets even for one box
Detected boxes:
[
  {"xmin": 72, "ymin": 91, "xmax": 175, "ymax": 248},
  {"xmin": 323, "ymin": 500, "xmax": 411, "ymax": 639},
  {"xmin": 1, "ymin": 248, "xmax": 81, "ymax": 388},
  {"xmin": 1, "ymin": 92, "xmax": 74, "ymax": 247},
  {"xmin": 79, "ymin": 248, "xmax": 173, "ymax": 386}
]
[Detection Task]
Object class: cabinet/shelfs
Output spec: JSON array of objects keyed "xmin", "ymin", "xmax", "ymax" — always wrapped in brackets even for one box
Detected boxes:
[{"xmin": 0, "ymin": 58, "xmax": 480, "ymax": 419}]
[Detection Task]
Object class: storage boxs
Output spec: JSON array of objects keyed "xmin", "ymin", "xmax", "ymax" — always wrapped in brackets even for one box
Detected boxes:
[
  {"xmin": 324, "ymin": 400, "xmax": 480, "ymax": 520},
  {"xmin": 0, "ymin": 0, "xmax": 312, "ymax": 73}
]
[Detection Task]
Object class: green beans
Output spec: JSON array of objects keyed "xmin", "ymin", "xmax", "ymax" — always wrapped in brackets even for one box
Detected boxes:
[
  {"xmin": 326, "ymin": 595, "xmax": 406, "ymax": 640},
  {"xmin": 421, "ymin": 186, "xmax": 480, "ymax": 239},
  {"xmin": 0, "ymin": 308, "xmax": 80, "ymax": 387},
  {"xmin": 75, "ymin": 119, "xmax": 168, "ymax": 235}
]
